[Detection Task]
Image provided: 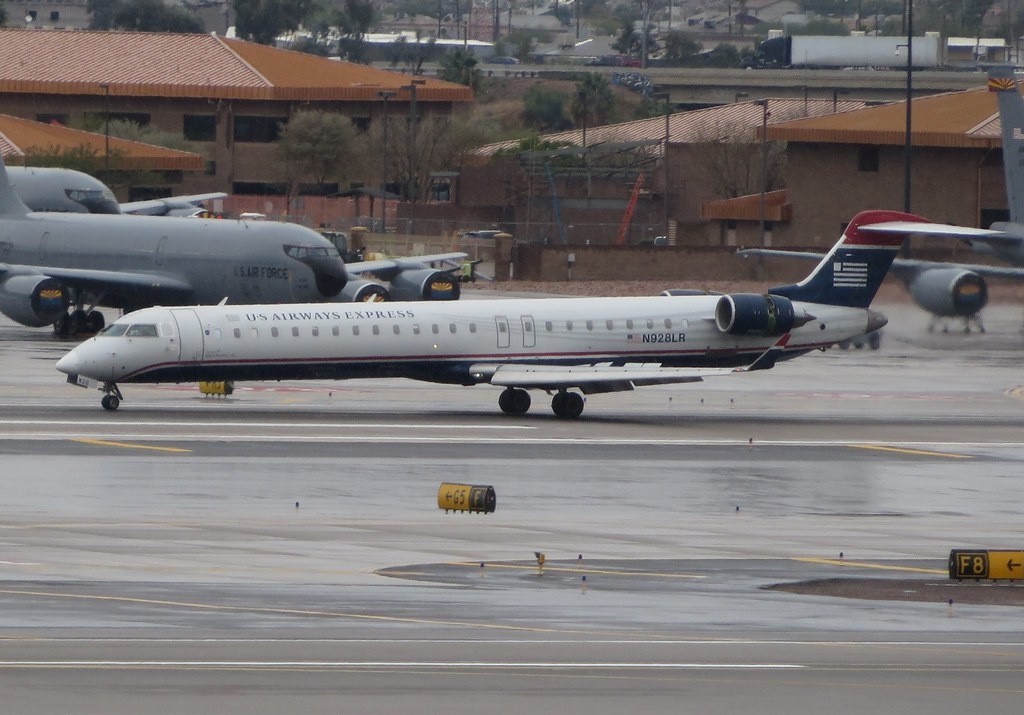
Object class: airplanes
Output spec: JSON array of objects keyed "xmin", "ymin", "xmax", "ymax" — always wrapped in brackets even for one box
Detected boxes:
[
  {"xmin": 737, "ymin": 250, "xmax": 1024, "ymax": 337},
  {"xmin": 858, "ymin": 62, "xmax": 1024, "ymax": 269},
  {"xmin": 55, "ymin": 211, "xmax": 930, "ymax": 422},
  {"xmin": 2, "ymin": 152, "xmax": 481, "ymax": 338}
]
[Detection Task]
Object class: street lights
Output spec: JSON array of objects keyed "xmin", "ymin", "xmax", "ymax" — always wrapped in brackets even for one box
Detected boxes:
[
  {"xmin": 578, "ymin": 90, "xmax": 587, "ymax": 165},
  {"xmin": 654, "ymin": 91, "xmax": 669, "ymax": 244},
  {"xmin": 754, "ymin": 99, "xmax": 767, "ymax": 280},
  {"xmin": 833, "ymin": 87, "xmax": 849, "ymax": 112},
  {"xmin": 791, "ymin": 84, "xmax": 808, "ymax": 119},
  {"xmin": 734, "ymin": 90, "xmax": 748, "ymax": 102}
]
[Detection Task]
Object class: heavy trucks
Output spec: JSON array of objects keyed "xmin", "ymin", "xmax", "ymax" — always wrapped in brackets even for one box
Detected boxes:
[{"xmin": 737, "ymin": 33, "xmax": 946, "ymax": 70}]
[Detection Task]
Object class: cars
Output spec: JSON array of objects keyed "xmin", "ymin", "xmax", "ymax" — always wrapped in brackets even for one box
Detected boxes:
[{"xmin": 486, "ymin": 56, "xmax": 522, "ymax": 64}]
